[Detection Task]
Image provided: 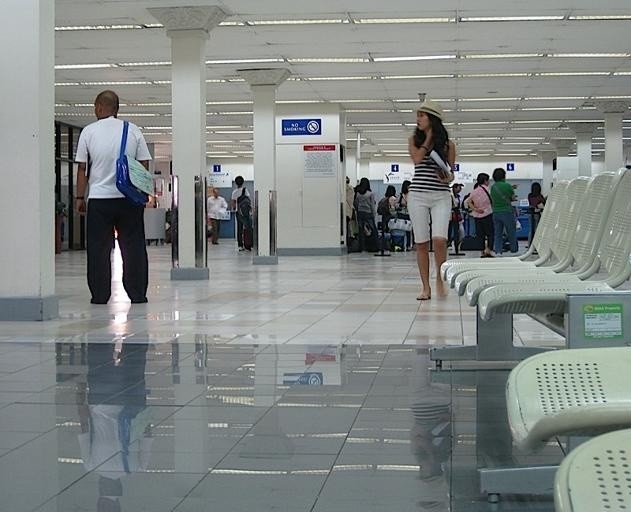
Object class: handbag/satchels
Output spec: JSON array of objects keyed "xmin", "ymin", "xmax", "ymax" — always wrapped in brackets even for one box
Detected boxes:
[
  {"xmin": 452, "ymin": 212, "xmax": 463, "ymax": 223},
  {"xmin": 115, "ymin": 153, "xmax": 155, "ymax": 208},
  {"xmin": 459, "ymin": 233, "xmax": 486, "ymax": 251}
]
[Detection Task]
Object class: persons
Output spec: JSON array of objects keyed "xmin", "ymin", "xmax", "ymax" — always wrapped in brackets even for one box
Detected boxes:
[
  {"xmin": 231, "ymin": 175, "xmax": 254, "ymax": 253},
  {"xmin": 73, "ymin": 90, "xmax": 156, "ymax": 305},
  {"xmin": 206, "ymin": 187, "xmax": 229, "ymax": 245},
  {"xmin": 76, "ymin": 315, "xmax": 152, "ymax": 511},
  {"xmin": 345, "ymin": 167, "xmax": 549, "ymax": 257},
  {"xmin": 407, "ymin": 98, "xmax": 459, "ymax": 302},
  {"xmin": 407, "ymin": 334, "xmax": 458, "ymax": 491}
]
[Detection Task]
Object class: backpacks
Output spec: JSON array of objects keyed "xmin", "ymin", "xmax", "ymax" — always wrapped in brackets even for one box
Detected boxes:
[
  {"xmin": 236, "ymin": 187, "xmax": 252, "ymax": 219},
  {"xmin": 376, "ymin": 197, "xmax": 391, "ymax": 215}
]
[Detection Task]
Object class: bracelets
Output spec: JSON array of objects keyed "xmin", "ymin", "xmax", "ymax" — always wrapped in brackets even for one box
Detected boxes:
[
  {"xmin": 420, "ymin": 144, "xmax": 429, "ymax": 153},
  {"xmin": 77, "ymin": 197, "xmax": 85, "ymax": 200}
]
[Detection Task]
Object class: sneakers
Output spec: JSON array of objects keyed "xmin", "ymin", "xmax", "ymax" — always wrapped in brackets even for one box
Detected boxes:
[{"xmin": 238, "ymin": 246, "xmax": 251, "ymax": 252}]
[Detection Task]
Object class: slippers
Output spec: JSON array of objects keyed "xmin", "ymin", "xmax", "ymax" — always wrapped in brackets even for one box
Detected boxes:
[{"xmin": 480, "ymin": 253, "xmax": 496, "ymax": 258}]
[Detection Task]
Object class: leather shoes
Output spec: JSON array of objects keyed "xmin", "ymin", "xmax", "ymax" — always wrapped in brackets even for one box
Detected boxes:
[{"xmin": 416, "ymin": 287, "xmax": 432, "ymax": 301}]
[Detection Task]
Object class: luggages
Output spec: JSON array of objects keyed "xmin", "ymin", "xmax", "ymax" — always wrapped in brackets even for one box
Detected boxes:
[{"xmin": 347, "ymin": 218, "xmax": 413, "ymax": 253}]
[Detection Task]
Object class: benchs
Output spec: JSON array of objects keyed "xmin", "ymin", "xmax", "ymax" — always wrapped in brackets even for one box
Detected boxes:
[{"xmin": 428, "ymin": 165, "xmax": 631, "ymax": 511}]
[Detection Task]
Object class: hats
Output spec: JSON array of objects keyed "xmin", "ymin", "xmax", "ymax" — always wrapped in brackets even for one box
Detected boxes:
[
  {"xmin": 452, "ymin": 184, "xmax": 464, "ymax": 188},
  {"xmin": 415, "ymin": 99, "xmax": 445, "ymax": 122}
]
